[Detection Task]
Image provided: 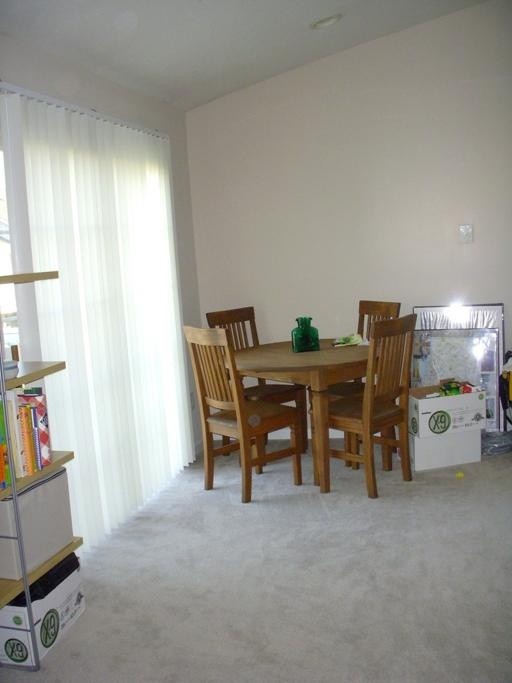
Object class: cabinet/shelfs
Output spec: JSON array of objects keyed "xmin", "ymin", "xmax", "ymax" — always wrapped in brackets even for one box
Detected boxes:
[{"xmin": 0, "ymin": 269, "xmax": 85, "ymax": 674}]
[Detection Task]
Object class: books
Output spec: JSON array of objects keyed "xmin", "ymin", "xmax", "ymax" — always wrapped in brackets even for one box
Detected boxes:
[
  {"xmin": 334, "ymin": 333, "xmax": 363, "ymax": 347},
  {"xmin": 1, "ymin": 385, "xmax": 52, "ymax": 491},
  {"xmin": 357, "ymin": 337, "xmax": 370, "ymax": 347}
]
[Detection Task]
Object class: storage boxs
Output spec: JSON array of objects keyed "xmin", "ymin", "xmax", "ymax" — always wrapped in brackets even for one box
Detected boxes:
[
  {"xmin": 0, "ymin": 466, "xmax": 86, "ymax": 667},
  {"xmin": 394, "ymin": 380, "xmax": 487, "ymax": 473}
]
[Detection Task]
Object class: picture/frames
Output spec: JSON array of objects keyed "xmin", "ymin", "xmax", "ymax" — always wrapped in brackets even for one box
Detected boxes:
[{"xmin": 411, "ymin": 302, "xmax": 505, "ymax": 432}]
